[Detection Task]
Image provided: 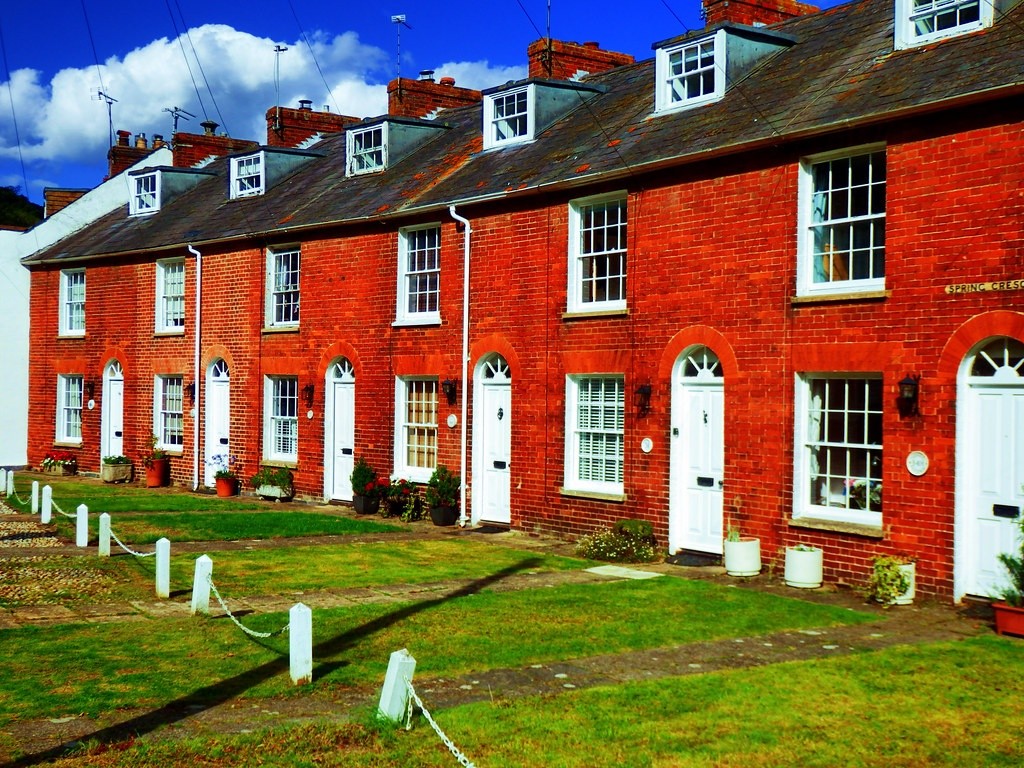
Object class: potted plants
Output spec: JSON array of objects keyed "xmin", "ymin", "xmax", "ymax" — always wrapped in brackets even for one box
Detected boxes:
[
  {"xmin": 724, "ymin": 517, "xmax": 761, "ymax": 577},
  {"xmin": 784, "ymin": 543, "xmax": 823, "ymax": 589},
  {"xmin": 249, "ymin": 465, "xmax": 293, "ymax": 498},
  {"xmin": 989, "ymin": 484, "xmax": 1024, "ymax": 640},
  {"xmin": 103, "ymin": 455, "xmax": 128, "ymax": 465},
  {"xmin": 140, "ymin": 433, "xmax": 168, "ymax": 488},
  {"xmin": 350, "ymin": 455, "xmax": 381, "ymax": 515},
  {"xmin": 425, "ymin": 464, "xmax": 462, "ymax": 526}
]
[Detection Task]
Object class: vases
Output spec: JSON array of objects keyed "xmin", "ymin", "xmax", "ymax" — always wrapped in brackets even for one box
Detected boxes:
[
  {"xmin": 43, "ymin": 464, "xmax": 74, "ymax": 477},
  {"xmin": 875, "ymin": 563, "xmax": 915, "ymax": 605},
  {"xmin": 214, "ymin": 477, "xmax": 237, "ymax": 497},
  {"xmin": 388, "ymin": 498, "xmax": 422, "ymax": 517},
  {"xmin": 101, "ymin": 464, "xmax": 132, "ymax": 482}
]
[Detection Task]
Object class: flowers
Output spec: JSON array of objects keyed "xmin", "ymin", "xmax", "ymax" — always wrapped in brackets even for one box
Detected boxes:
[
  {"xmin": 365, "ymin": 478, "xmax": 427, "ymax": 522},
  {"xmin": 858, "ymin": 555, "xmax": 917, "ymax": 611},
  {"xmin": 202, "ymin": 454, "xmax": 240, "ymax": 479},
  {"xmin": 38, "ymin": 452, "xmax": 78, "ymax": 472}
]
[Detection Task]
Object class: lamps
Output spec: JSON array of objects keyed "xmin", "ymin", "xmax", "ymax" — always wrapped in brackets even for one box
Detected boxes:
[
  {"xmin": 184, "ymin": 382, "xmax": 195, "ymax": 405},
  {"xmin": 83, "ymin": 381, "xmax": 94, "ymax": 399},
  {"xmin": 895, "ymin": 372, "xmax": 922, "ymax": 420},
  {"xmin": 632, "ymin": 380, "xmax": 652, "ymax": 418},
  {"xmin": 301, "ymin": 383, "xmax": 314, "ymax": 409},
  {"xmin": 441, "ymin": 376, "xmax": 457, "ymax": 406}
]
